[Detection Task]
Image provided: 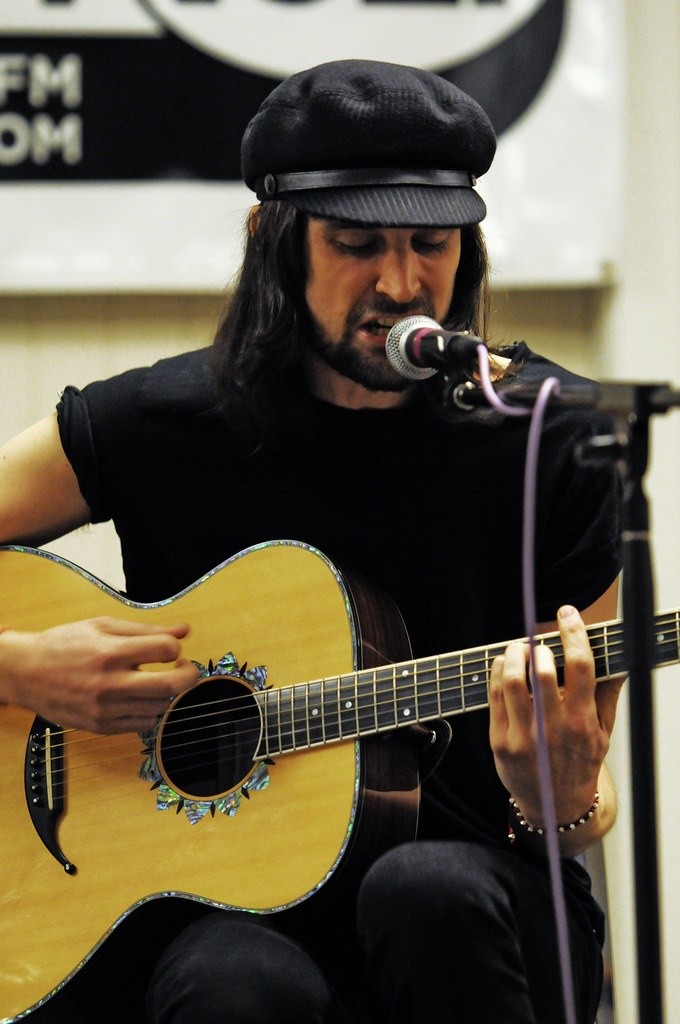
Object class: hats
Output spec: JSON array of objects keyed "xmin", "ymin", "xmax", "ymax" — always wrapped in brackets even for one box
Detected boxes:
[{"xmin": 242, "ymin": 60, "xmax": 496, "ymax": 229}]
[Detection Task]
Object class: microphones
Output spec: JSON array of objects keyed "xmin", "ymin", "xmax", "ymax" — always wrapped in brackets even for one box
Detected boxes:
[{"xmin": 385, "ymin": 315, "xmax": 483, "ymax": 380}]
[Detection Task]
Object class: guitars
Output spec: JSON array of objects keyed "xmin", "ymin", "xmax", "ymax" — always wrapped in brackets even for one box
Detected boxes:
[{"xmin": 0, "ymin": 535, "xmax": 680, "ymax": 1024}]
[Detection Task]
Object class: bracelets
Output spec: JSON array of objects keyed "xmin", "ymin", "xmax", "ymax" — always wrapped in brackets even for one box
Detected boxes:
[{"xmin": 509, "ymin": 790, "xmax": 599, "ymax": 835}]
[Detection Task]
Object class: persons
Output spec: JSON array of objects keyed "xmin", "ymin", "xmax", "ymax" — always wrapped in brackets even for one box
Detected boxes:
[{"xmin": 0, "ymin": 59, "xmax": 626, "ymax": 1023}]
